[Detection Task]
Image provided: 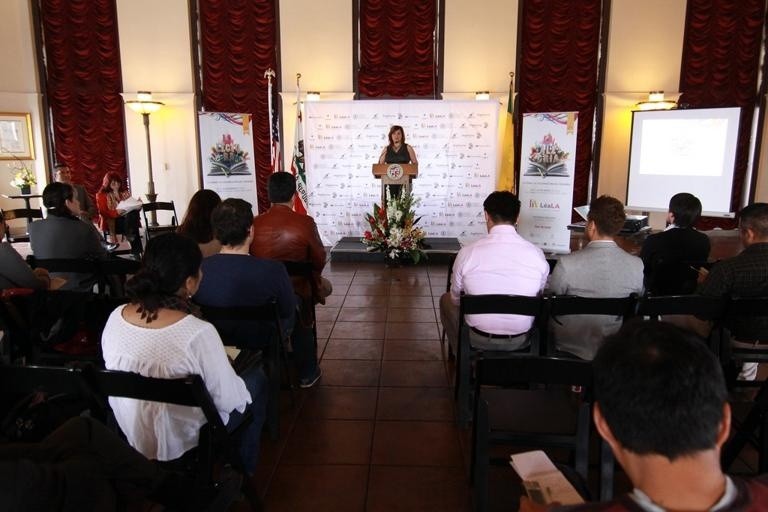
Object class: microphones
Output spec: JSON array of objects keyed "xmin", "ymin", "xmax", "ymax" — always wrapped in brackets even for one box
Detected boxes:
[{"xmin": 378, "ymin": 141, "xmax": 399, "ymax": 164}]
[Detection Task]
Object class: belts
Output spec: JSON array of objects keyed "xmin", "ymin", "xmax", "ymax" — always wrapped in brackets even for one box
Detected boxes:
[{"xmin": 469, "ymin": 326, "xmax": 527, "ymax": 339}]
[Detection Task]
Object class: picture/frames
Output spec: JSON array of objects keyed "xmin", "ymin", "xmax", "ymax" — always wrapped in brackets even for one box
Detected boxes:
[{"xmin": 0, "ymin": 111, "xmax": 38, "ymax": 159}]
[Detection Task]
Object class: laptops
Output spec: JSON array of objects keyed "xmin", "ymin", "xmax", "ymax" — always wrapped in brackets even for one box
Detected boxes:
[{"xmin": 566, "ymin": 205, "xmax": 590, "ymax": 228}]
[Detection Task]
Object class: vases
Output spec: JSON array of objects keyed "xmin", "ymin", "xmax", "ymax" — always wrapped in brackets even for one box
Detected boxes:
[{"xmin": 20, "ymin": 186, "xmax": 31, "ymax": 195}]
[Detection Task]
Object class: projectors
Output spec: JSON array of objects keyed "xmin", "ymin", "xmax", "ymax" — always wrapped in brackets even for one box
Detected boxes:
[{"xmin": 621, "ymin": 214, "xmax": 648, "ymax": 232}]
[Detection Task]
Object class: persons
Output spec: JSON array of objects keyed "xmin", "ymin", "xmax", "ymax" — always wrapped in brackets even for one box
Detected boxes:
[
  {"xmin": 181, "ymin": 188, "xmax": 321, "ymax": 389},
  {"xmin": 249, "ymin": 171, "xmax": 332, "ymax": 305},
  {"xmin": 638, "ymin": 193, "xmax": 710, "ymax": 296},
  {"xmin": 95, "ymin": 172, "xmax": 144, "ymax": 260},
  {"xmin": 439, "ymin": 191, "xmax": 551, "ymax": 364},
  {"xmin": 378, "ymin": 126, "xmax": 417, "ymax": 164},
  {"xmin": 100, "ymin": 233, "xmax": 279, "ymax": 486},
  {"xmin": 519, "ymin": 318, "xmax": 768, "ymax": 512},
  {"xmin": 0, "ymin": 182, "xmax": 143, "ymax": 352},
  {"xmin": 693, "ymin": 203, "xmax": 768, "ymax": 393},
  {"xmin": 550, "ymin": 196, "xmax": 645, "ymax": 384}
]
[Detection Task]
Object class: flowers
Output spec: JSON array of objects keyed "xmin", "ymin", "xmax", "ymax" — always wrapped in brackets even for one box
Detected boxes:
[
  {"xmin": 8, "ymin": 159, "xmax": 38, "ymax": 186},
  {"xmin": 359, "ymin": 188, "xmax": 434, "ymax": 266}
]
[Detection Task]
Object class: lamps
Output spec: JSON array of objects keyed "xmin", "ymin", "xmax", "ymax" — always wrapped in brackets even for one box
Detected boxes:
[
  {"xmin": 636, "ymin": 89, "xmax": 680, "ymax": 112},
  {"xmin": 125, "ymin": 90, "xmax": 164, "ymax": 226}
]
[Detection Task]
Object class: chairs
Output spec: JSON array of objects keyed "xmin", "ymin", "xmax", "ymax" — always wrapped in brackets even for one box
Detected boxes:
[
  {"xmin": 432, "ymin": 253, "xmax": 768, "ymax": 511},
  {"xmin": 0, "ymin": 201, "xmax": 306, "ymax": 512}
]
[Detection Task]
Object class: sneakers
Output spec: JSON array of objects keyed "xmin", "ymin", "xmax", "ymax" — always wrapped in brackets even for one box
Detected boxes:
[{"xmin": 299, "ymin": 364, "xmax": 323, "ymax": 389}]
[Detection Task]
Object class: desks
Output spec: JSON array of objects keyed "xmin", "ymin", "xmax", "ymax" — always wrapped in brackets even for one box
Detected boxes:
[{"xmin": 1, "ymin": 194, "xmax": 44, "ymax": 236}]
